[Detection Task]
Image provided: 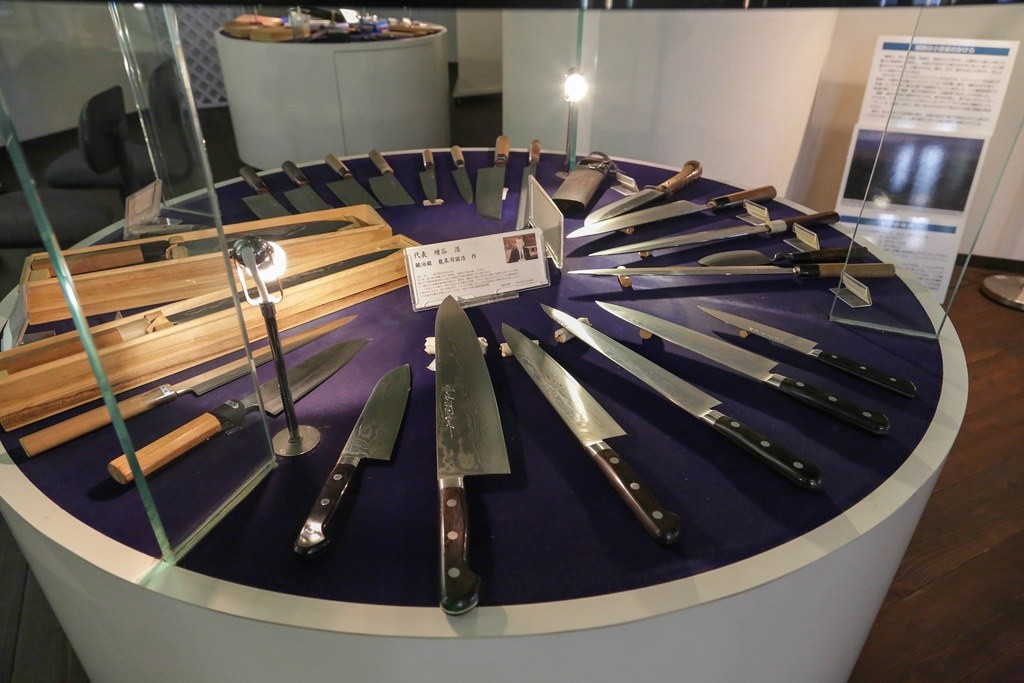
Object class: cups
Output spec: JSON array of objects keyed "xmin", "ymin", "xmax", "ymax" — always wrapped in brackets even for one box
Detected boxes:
[{"xmin": 291, "ymin": 14, "xmax": 311, "ymax": 40}]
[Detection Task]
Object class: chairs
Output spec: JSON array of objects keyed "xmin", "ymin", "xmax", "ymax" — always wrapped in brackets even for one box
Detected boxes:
[
  {"xmin": 0, "ymin": 84, "xmax": 138, "ymax": 248},
  {"xmin": 48, "ymin": 56, "xmax": 195, "ymax": 191}
]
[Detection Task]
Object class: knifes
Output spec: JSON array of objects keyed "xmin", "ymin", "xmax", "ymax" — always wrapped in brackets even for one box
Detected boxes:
[
  {"xmin": 696, "ymin": 305, "xmax": 917, "ymax": 397},
  {"xmin": 295, "ymin": 364, "xmax": 410, "ymax": 554},
  {"xmin": 451, "ymin": 145, "xmax": 473, "ymax": 204},
  {"xmin": 239, "ymin": 166, "xmax": 291, "ymax": 220},
  {"xmin": 30, "ymin": 220, "xmax": 353, "ymax": 277},
  {"xmin": 325, "ymin": 154, "xmax": 382, "ymax": 209},
  {"xmin": 108, "ymin": 340, "xmax": 366, "ymax": 483},
  {"xmin": 19, "ymin": 316, "xmax": 357, "ymax": 457},
  {"xmin": 281, "ymin": 161, "xmax": 330, "ymax": 214},
  {"xmin": 540, "ymin": 303, "xmax": 824, "ymax": 490},
  {"xmin": 476, "ymin": 137, "xmax": 509, "ymax": 219},
  {"xmin": 419, "ymin": 150, "xmax": 437, "ymax": 204},
  {"xmin": 0, "ymin": 249, "xmax": 400, "ymax": 376},
  {"xmin": 596, "ymin": 301, "xmax": 891, "ymax": 435},
  {"xmin": 502, "ymin": 323, "xmax": 682, "ymax": 545},
  {"xmin": 516, "ymin": 139, "xmax": 541, "ymax": 231},
  {"xmin": 563, "ymin": 161, "xmax": 895, "ymax": 277},
  {"xmin": 435, "ymin": 296, "xmax": 511, "ymax": 616},
  {"xmin": 369, "ymin": 150, "xmax": 416, "ymax": 207}
]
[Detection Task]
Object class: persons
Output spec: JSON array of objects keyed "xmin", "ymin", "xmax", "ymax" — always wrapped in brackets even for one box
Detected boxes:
[{"xmin": 509, "ymin": 236, "xmax": 530, "ymax": 262}]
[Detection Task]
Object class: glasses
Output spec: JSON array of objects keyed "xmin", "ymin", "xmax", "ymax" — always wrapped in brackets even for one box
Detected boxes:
[{"xmin": 515, "ymin": 242, "xmax": 523, "ymax": 245}]
